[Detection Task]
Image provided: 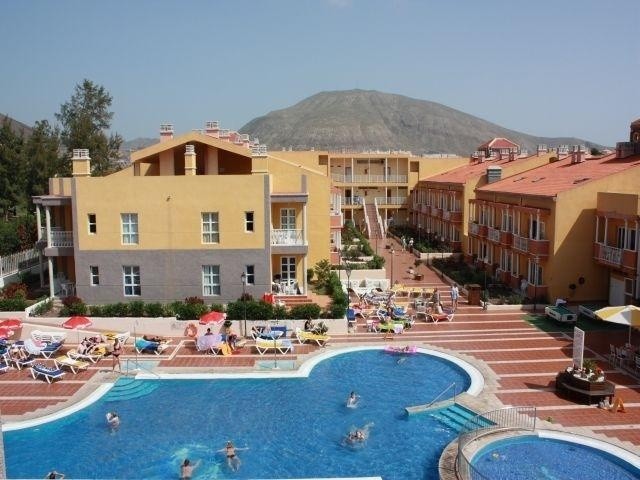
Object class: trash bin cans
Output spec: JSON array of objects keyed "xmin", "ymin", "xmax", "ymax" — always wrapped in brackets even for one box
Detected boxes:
[{"xmin": 468, "ymin": 284, "xmax": 481, "ymax": 305}]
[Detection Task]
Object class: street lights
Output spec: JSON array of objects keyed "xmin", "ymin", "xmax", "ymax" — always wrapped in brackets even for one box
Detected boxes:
[
  {"xmin": 373, "ymin": 227, "xmax": 381, "ymax": 256},
  {"xmin": 388, "ymin": 240, "xmax": 396, "ymax": 290},
  {"xmin": 483, "ymin": 254, "xmax": 490, "ymax": 310},
  {"xmin": 239, "ymin": 270, "xmax": 253, "ymax": 339},
  {"xmin": 405, "ymin": 214, "xmax": 448, "ymax": 279},
  {"xmin": 344, "ymin": 262, "xmax": 355, "ymax": 328},
  {"xmin": 391, "ymin": 209, "xmax": 397, "ymax": 239},
  {"xmin": 532, "ymin": 254, "xmax": 541, "ymax": 313},
  {"xmin": 336, "ymin": 246, "xmax": 344, "ymax": 282},
  {"xmin": 361, "ymin": 215, "xmax": 366, "ymax": 236}
]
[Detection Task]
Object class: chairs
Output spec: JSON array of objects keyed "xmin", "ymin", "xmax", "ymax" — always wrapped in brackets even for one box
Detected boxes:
[
  {"xmin": 210, "ymin": 326, "xmax": 331, "ymax": 356},
  {"xmin": 610, "ymin": 344, "xmax": 634, "ymax": 369},
  {"xmin": 348, "ymin": 287, "xmax": 455, "ymax": 333},
  {"xmin": 0, "ymin": 331, "xmax": 173, "ymax": 384}
]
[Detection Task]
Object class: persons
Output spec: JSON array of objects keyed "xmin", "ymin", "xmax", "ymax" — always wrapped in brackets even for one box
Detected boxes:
[
  {"xmin": 106, "ymin": 412, "xmax": 119, "ymax": 436},
  {"xmin": 217, "ymin": 441, "xmax": 248, "ymax": 471},
  {"xmin": 228, "ymin": 333, "xmax": 237, "ymax": 351},
  {"xmin": 252, "ymin": 326, "xmax": 280, "ymax": 340},
  {"xmin": 142, "ymin": 335, "xmax": 166, "ymax": 351},
  {"xmin": 361, "ymin": 286, "xmax": 440, "ymax": 341},
  {"xmin": 45, "ymin": 471, "xmax": 64, "ymax": 479},
  {"xmin": 304, "ymin": 317, "xmax": 328, "ymax": 335},
  {"xmin": 204, "ymin": 328, "xmax": 213, "ymax": 355},
  {"xmin": 346, "ymin": 391, "xmax": 359, "ymax": 408},
  {"xmin": 348, "ymin": 430, "xmax": 365, "ymax": 443},
  {"xmin": 388, "ymin": 236, "xmax": 416, "ymax": 257},
  {"xmin": 78, "ymin": 335, "xmax": 107, "ymax": 355},
  {"xmin": 392, "ymin": 346, "xmax": 409, "ymax": 351},
  {"xmin": 0, "ymin": 346, "xmax": 30, "ymax": 369},
  {"xmin": 451, "ymin": 284, "xmax": 459, "ymax": 310},
  {"xmin": 180, "ymin": 458, "xmax": 202, "ymax": 480},
  {"xmin": 113, "ymin": 338, "xmax": 124, "ymax": 372}
]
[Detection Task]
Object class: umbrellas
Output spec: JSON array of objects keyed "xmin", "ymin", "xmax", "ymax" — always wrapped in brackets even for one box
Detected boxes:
[
  {"xmin": 0, "ymin": 318, "xmax": 22, "ymax": 339},
  {"xmin": 593, "ymin": 305, "xmax": 640, "ymax": 344},
  {"xmin": 199, "ymin": 312, "xmax": 227, "ymax": 326},
  {"xmin": 62, "ymin": 317, "xmax": 92, "ymax": 329}
]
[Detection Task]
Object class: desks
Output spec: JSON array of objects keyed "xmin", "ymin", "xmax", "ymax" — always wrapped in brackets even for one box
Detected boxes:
[{"xmin": 196, "ymin": 334, "xmax": 225, "ymax": 355}]
